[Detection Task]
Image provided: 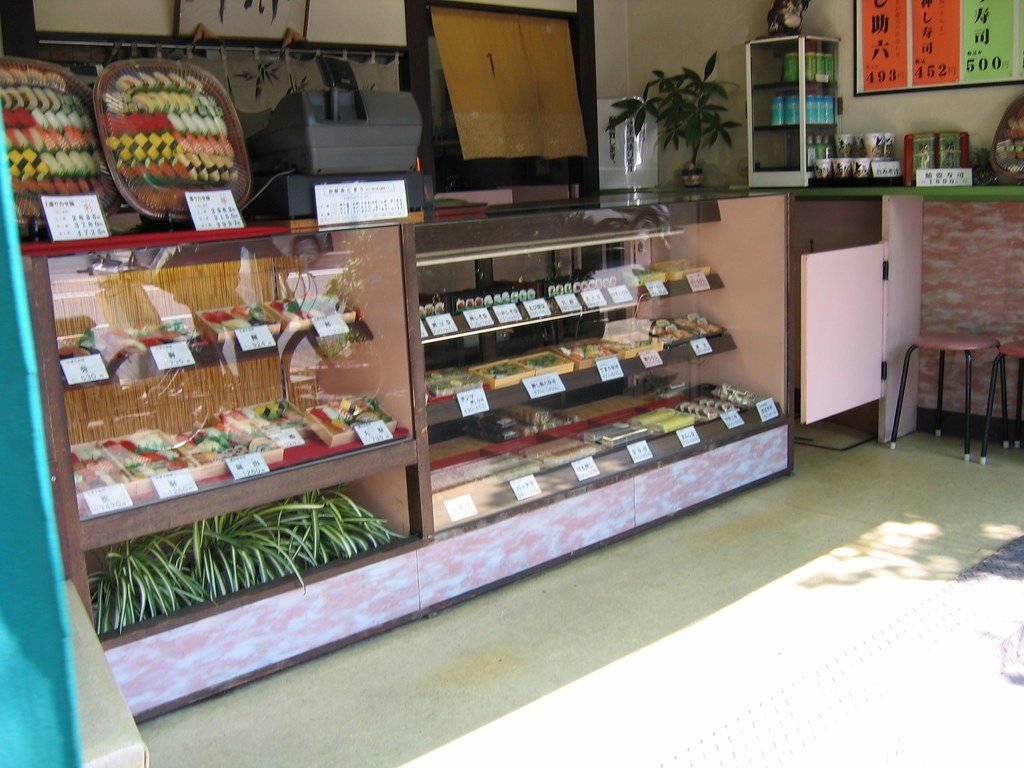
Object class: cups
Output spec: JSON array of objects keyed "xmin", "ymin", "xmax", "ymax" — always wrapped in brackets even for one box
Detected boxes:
[
  {"xmin": 854, "ymin": 134, "xmax": 865, "ymax": 156},
  {"xmin": 865, "ymin": 133, "xmax": 884, "ymax": 157},
  {"xmin": 852, "ymin": 157, "xmax": 872, "ymax": 179},
  {"xmin": 835, "ymin": 134, "xmax": 854, "ymax": 157},
  {"xmin": 883, "ymin": 132, "xmax": 896, "ymax": 160},
  {"xmin": 813, "ymin": 159, "xmax": 832, "ymax": 178},
  {"xmin": 833, "ymin": 157, "xmax": 852, "ymax": 178}
]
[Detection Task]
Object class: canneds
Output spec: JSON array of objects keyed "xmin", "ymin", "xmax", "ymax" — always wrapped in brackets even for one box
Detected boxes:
[{"xmin": 771, "ymin": 50, "xmax": 834, "ymax": 124}]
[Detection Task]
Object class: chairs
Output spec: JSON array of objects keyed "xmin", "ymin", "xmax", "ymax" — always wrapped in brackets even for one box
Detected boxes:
[
  {"xmin": 889, "ymin": 336, "xmax": 1000, "ymax": 461},
  {"xmin": 979, "ymin": 338, "xmax": 1024, "ymax": 464}
]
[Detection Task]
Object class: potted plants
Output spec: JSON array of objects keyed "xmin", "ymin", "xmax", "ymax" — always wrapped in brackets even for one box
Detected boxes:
[{"xmin": 605, "ymin": 49, "xmax": 741, "ymax": 190}]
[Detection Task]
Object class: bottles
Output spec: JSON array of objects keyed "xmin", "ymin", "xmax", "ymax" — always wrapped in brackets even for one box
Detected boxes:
[
  {"xmin": 807, "ymin": 135, "xmax": 814, "ymax": 169},
  {"xmin": 823, "ymin": 136, "xmax": 834, "ymax": 158},
  {"xmin": 814, "ymin": 135, "xmax": 824, "ymax": 159}
]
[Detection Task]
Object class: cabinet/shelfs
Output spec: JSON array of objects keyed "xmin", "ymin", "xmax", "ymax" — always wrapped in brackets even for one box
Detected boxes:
[
  {"xmin": 744, "ymin": 32, "xmax": 842, "ymax": 188},
  {"xmin": 16, "ymin": 193, "xmax": 791, "ymax": 723}
]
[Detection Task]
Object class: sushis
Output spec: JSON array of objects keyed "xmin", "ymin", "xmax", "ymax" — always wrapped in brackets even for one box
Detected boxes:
[
  {"xmin": 418, "ymin": 273, "xmax": 739, "ymax": 423},
  {"xmin": 0, "ymin": 68, "xmax": 241, "ymax": 222},
  {"xmin": 62, "ymin": 298, "xmax": 388, "ymax": 490}
]
